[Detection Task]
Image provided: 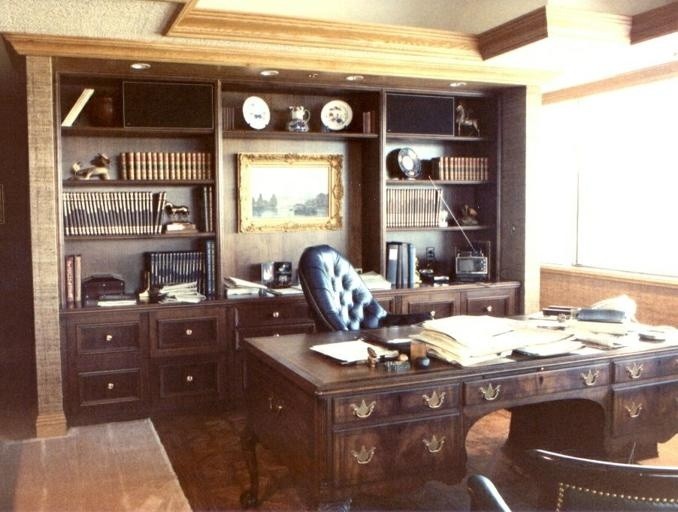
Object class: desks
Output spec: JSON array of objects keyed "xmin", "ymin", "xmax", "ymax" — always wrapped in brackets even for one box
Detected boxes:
[{"xmin": 241, "ymin": 311, "xmax": 677, "ymax": 511}]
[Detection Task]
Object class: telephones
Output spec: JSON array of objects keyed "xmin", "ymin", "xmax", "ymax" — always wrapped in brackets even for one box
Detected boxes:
[{"xmin": 638, "ymin": 325, "xmax": 671, "ymax": 341}]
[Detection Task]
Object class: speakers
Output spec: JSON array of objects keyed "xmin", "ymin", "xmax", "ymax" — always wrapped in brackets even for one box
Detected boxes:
[
  {"xmin": 386, "ymin": 91, "xmax": 457, "ymax": 137},
  {"xmin": 121, "ymin": 79, "xmax": 216, "ymax": 131}
]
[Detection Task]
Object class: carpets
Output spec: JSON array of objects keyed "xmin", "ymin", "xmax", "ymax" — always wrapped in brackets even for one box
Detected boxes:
[{"xmin": 0, "ymin": 419, "xmax": 195, "ymax": 512}]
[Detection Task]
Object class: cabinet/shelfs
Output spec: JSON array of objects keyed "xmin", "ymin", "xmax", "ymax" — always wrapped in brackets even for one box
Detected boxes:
[
  {"xmin": 54, "ymin": 71, "xmax": 219, "ymax": 307},
  {"xmin": 60, "ymin": 308, "xmax": 229, "ymax": 428},
  {"xmin": 403, "ymin": 283, "xmax": 518, "ymax": 327},
  {"xmin": 220, "ymin": 80, "xmax": 384, "ymax": 139},
  {"xmin": 230, "ymin": 291, "xmax": 404, "ymax": 411},
  {"xmin": 362, "ymin": 91, "xmax": 499, "ymax": 282}
]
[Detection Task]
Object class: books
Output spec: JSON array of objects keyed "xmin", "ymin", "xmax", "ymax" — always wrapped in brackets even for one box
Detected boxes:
[
  {"xmin": 224, "ymin": 273, "xmax": 388, "ymax": 297},
  {"xmin": 385, "ymin": 143, "xmax": 488, "ymax": 287},
  {"xmin": 309, "ymin": 294, "xmax": 678, "ymax": 366},
  {"xmin": 62, "ymin": 81, "xmax": 217, "ymax": 305}
]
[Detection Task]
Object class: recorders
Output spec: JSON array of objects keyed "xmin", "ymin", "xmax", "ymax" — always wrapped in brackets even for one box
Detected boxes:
[{"xmin": 455, "ymin": 249, "xmax": 488, "ymax": 283}]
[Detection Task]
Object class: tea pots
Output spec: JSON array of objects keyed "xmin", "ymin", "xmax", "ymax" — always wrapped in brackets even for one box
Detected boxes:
[{"xmin": 287, "ymin": 106, "xmax": 311, "ymax": 133}]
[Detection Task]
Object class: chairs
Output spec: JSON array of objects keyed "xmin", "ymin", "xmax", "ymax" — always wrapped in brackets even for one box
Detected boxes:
[
  {"xmin": 464, "ymin": 448, "xmax": 677, "ymax": 511},
  {"xmin": 297, "ymin": 245, "xmax": 427, "ymax": 330}
]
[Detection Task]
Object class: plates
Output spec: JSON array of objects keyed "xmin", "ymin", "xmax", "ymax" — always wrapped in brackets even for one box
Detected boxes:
[
  {"xmin": 242, "ymin": 95, "xmax": 271, "ymax": 130},
  {"xmin": 397, "ymin": 148, "xmax": 421, "ymax": 178},
  {"xmin": 320, "ymin": 100, "xmax": 353, "ymax": 131}
]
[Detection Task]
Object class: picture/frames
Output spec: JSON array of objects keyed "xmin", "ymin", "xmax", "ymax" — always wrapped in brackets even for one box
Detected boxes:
[{"xmin": 235, "ymin": 151, "xmax": 346, "ymax": 236}]
